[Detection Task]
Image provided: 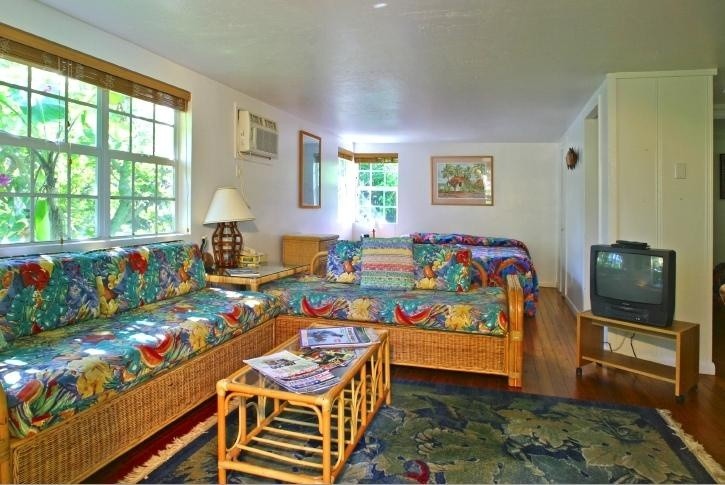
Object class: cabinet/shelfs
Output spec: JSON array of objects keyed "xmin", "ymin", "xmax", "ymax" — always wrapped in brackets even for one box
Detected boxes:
[
  {"xmin": 283, "ymin": 235, "xmax": 339, "ymax": 273},
  {"xmin": 575, "ymin": 310, "xmax": 700, "ymax": 402}
]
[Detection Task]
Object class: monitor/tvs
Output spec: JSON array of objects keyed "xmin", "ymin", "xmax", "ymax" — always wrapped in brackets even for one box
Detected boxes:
[{"xmin": 590, "ymin": 244, "xmax": 677, "ymax": 328}]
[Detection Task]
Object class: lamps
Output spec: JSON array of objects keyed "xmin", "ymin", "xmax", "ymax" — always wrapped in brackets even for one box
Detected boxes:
[{"xmin": 203, "ymin": 188, "xmax": 255, "ymax": 266}]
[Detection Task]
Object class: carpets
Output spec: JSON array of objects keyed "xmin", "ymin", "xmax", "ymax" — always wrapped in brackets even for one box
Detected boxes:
[{"xmin": 118, "ymin": 379, "xmax": 725, "ymax": 484}]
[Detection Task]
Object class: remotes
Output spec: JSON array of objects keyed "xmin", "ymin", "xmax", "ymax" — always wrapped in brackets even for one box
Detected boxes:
[
  {"xmin": 611, "ymin": 244, "xmax": 646, "ymax": 250},
  {"xmin": 616, "ymin": 239, "xmax": 647, "ymax": 247}
]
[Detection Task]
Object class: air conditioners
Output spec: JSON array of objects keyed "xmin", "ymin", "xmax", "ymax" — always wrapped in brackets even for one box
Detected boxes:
[{"xmin": 239, "ymin": 109, "xmax": 280, "ymax": 157}]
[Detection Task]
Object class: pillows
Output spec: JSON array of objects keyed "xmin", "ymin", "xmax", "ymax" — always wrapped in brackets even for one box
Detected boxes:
[{"xmin": 360, "ymin": 238, "xmax": 414, "ymax": 290}]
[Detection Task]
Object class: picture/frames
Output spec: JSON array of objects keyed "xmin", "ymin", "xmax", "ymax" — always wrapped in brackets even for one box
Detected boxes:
[{"xmin": 431, "ymin": 156, "xmax": 493, "ymax": 206}]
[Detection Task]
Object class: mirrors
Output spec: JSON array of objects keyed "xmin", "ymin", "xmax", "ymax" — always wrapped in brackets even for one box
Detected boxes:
[{"xmin": 299, "ymin": 130, "xmax": 321, "ymax": 208}]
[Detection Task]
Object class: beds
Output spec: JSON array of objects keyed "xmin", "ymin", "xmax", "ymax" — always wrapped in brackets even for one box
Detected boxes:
[{"xmin": 410, "ymin": 234, "xmax": 538, "ymax": 317}]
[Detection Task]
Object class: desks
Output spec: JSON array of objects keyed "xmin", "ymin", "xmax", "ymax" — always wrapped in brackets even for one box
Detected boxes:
[{"xmin": 205, "ymin": 264, "xmax": 310, "ymax": 291}]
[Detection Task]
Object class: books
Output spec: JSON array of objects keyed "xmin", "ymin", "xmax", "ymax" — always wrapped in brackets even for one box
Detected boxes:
[
  {"xmin": 225, "ymin": 268, "xmax": 260, "ymax": 277},
  {"xmin": 243, "ymin": 326, "xmax": 380, "ymax": 394}
]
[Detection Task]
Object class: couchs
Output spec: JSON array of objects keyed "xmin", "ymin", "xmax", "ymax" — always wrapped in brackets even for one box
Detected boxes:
[
  {"xmin": 264, "ymin": 246, "xmax": 523, "ymax": 387},
  {"xmin": 0, "ymin": 239, "xmax": 277, "ymax": 484}
]
